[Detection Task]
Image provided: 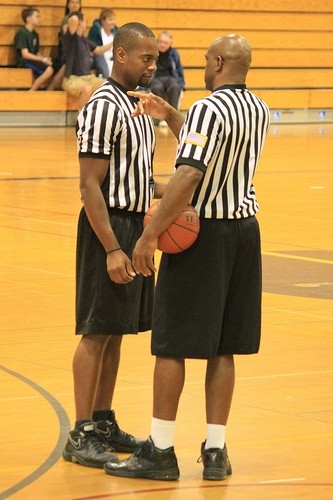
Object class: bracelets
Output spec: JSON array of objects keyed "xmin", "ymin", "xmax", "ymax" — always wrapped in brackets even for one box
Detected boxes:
[
  {"xmin": 43, "ymin": 56, "xmax": 48, "ymax": 63},
  {"xmin": 105, "ymin": 246, "xmax": 122, "ymax": 257}
]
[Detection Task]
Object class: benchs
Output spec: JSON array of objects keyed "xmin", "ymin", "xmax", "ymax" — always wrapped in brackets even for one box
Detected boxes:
[{"xmin": 0, "ymin": 0, "xmax": 332, "ymax": 127}]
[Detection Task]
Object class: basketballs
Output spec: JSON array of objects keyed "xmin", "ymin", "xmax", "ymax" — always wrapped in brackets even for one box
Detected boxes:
[{"xmin": 142, "ymin": 199, "xmax": 201, "ymax": 254}]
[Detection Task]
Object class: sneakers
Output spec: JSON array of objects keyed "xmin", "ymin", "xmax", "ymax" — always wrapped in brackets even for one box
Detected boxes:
[
  {"xmin": 62, "ymin": 421, "xmax": 119, "ymax": 468},
  {"xmin": 103, "ymin": 436, "xmax": 179, "ymax": 480},
  {"xmin": 197, "ymin": 439, "xmax": 232, "ymax": 480},
  {"xmin": 94, "ymin": 411, "xmax": 147, "ymax": 452}
]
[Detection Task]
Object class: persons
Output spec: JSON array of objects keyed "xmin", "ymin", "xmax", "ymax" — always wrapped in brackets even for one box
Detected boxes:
[
  {"xmin": 61, "ymin": 11, "xmax": 114, "ymax": 122},
  {"xmin": 141, "ymin": 29, "xmax": 186, "ymax": 126},
  {"xmin": 60, "ymin": 20, "xmax": 173, "ymax": 470},
  {"xmin": 86, "ymin": 8, "xmax": 121, "ymax": 81},
  {"xmin": 103, "ymin": 32, "xmax": 271, "ymax": 480},
  {"xmin": 45, "ymin": 0, "xmax": 83, "ymax": 92},
  {"xmin": 12, "ymin": 7, "xmax": 53, "ymax": 93}
]
